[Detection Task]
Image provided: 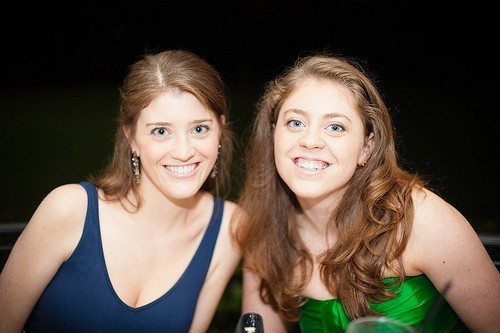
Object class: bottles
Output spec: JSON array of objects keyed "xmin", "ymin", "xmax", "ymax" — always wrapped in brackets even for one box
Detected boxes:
[{"xmin": 236, "ymin": 312, "xmax": 265, "ymax": 333}]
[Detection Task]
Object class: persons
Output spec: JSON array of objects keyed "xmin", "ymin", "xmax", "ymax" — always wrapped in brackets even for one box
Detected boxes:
[
  {"xmin": 238, "ymin": 53, "xmax": 500, "ymax": 333},
  {"xmin": 0, "ymin": 51, "xmax": 251, "ymax": 333}
]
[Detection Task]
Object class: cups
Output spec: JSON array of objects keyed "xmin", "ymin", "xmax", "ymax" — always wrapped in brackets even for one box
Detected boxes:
[{"xmin": 343, "ymin": 316, "xmax": 418, "ymax": 333}]
